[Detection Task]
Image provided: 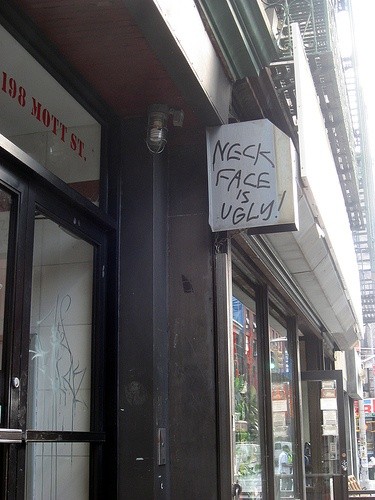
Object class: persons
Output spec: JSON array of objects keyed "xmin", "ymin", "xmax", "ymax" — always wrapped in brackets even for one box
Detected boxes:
[{"xmin": 279, "ymin": 444, "xmax": 293, "ymax": 491}]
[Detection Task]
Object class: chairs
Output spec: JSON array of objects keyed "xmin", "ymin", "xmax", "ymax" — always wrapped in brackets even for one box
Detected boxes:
[{"xmin": 348, "ymin": 475, "xmax": 372, "ymax": 500}]
